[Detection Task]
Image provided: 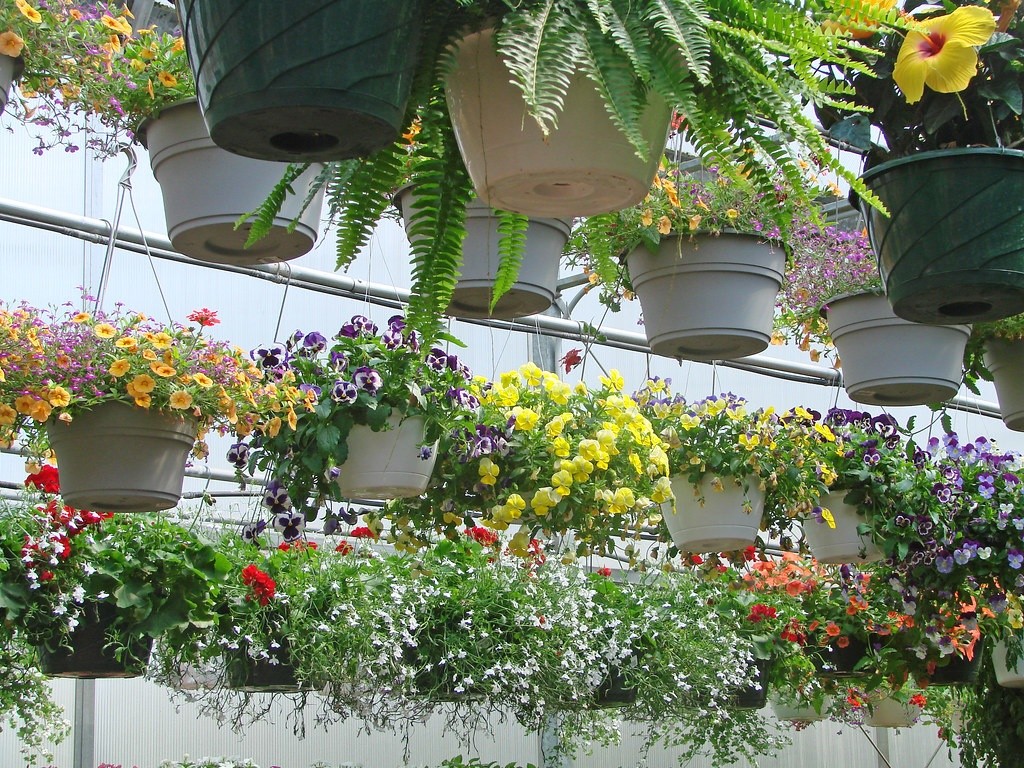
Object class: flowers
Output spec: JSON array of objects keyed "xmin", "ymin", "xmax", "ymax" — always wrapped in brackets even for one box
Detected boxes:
[
  {"xmin": 362, "ymin": 358, "xmax": 676, "ymax": 572},
  {"xmin": 209, "ymin": 527, "xmax": 370, "ymax": 688},
  {"xmin": 776, "ymin": 405, "xmax": 965, "ymax": 584},
  {"xmin": 631, "ymin": 375, "xmax": 849, "ymax": 536},
  {"xmin": 0, "ymin": 283, "xmax": 317, "ymax": 473},
  {"xmin": 0, "ymin": 464, "xmax": 233, "ymax": 744},
  {"xmin": 223, "ymin": 314, "xmax": 514, "ymax": 542}
]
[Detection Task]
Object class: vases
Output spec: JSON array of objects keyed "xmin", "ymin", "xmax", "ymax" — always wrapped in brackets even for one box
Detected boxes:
[
  {"xmin": 43, "ymin": 398, "xmax": 203, "ymax": 508},
  {"xmin": 334, "ymin": 406, "xmax": 442, "ymax": 500},
  {"xmin": 41, "ymin": 599, "xmax": 154, "ymax": 679},
  {"xmin": 382, "ymin": 528, "xmax": 1024, "ymax": 767},
  {"xmin": 219, "ymin": 608, "xmax": 346, "ymax": 691},
  {"xmin": 802, "ymin": 488, "xmax": 886, "ymax": 568},
  {"xmin": 494, "ymin": 490, "xmax": 550, "ymax": 524},
  {"xmin": 659, "ymin": 470, "xmax": 766, "ymax": 553},
  {"xmin": 0, "ymin": 1, "xmax": 1024, "ymax": 434}
]
